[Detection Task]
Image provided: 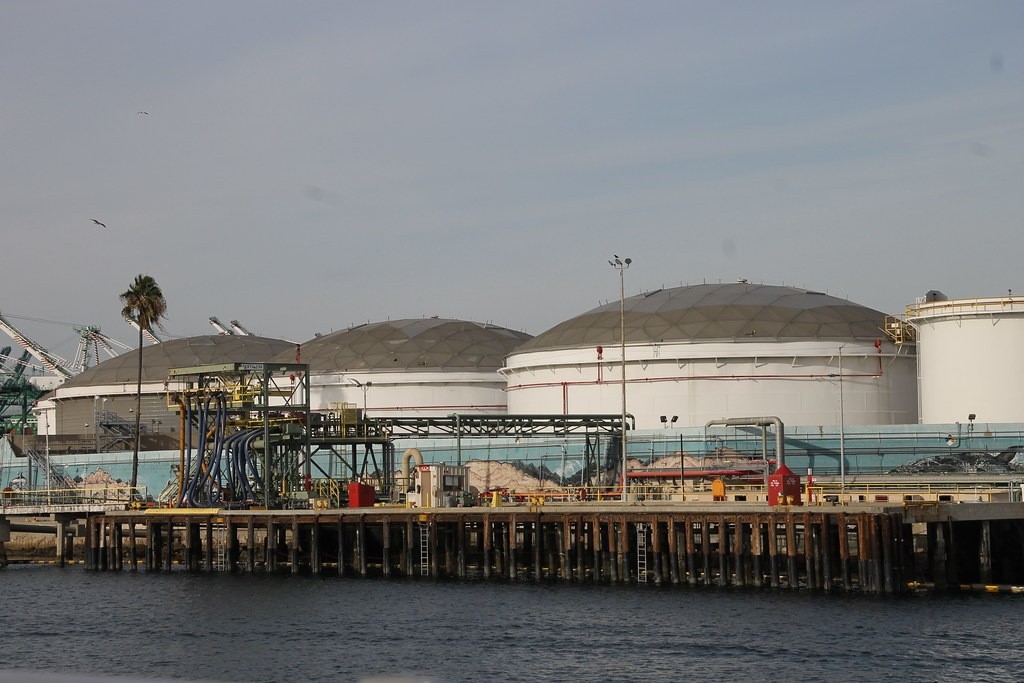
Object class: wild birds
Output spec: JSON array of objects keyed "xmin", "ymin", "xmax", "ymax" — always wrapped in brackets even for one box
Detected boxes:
[
  {"xmin": 89, "ymin": 219, "xmax": 107, "ymax": 228},
  {"xmin": 136, "ymin": 111, "xmax": 148, "ymax": 115}
]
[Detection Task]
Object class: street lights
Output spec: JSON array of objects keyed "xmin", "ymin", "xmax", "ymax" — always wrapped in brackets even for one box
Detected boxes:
[
  {"xmin": 33, "ymin": 411, "xmax": 51, "ymax": 507},
  {"xmin": 608, "ymin": 254, "xmax": 632, "ymax": 504}
]
